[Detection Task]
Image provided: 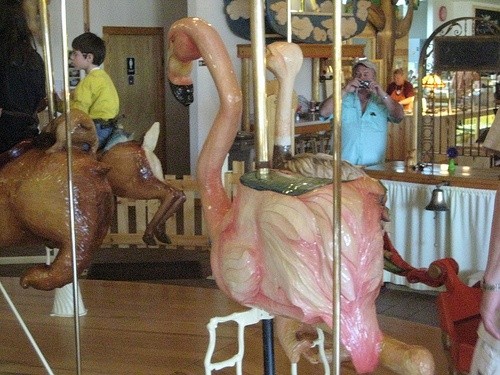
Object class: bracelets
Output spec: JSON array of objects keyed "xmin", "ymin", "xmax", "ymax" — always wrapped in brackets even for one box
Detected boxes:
[{"xmin": 382, "ymin": 94, "xmax": 388, "ymax": 100}]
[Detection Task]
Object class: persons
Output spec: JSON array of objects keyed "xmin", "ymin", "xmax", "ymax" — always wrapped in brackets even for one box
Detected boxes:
[
  {"xmin": 385, "ymin": 68, "xmax": 416, "ymax": 112},
  {"xmin": 0, "ymin": 0, "xmax": 45, "ymax": 157},
  {"xmin": 45, "ymin": 31, "xmax": 120, "ymax": 150},
  {"xmin": 319, "ymin": 59, "xmax": 404, "ymax": 167}
]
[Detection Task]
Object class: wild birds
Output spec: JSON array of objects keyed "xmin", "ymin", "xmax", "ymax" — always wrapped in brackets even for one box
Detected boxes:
[{"xmin": 168, "ymin": 17, "xmax": 459, "ymax": 375}]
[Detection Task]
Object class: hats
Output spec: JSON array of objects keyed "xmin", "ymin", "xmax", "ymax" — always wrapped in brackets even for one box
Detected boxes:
[{"xmin": 352, "ymin": 59, "xmax": 377, "ymax": 73}]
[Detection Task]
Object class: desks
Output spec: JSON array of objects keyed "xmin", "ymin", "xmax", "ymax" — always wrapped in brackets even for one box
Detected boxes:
[{"xmin": 367, "ymin": 161, "xmax": 500, "ymax": 292}]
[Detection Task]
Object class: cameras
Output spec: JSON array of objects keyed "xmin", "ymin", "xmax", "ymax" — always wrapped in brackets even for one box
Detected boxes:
[{"xmin": 358, "ymin": 80, "xmax": 369, "ymax": 88}]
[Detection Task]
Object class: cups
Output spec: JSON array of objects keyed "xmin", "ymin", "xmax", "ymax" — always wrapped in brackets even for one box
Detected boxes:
[{"xmin": 309, "ymin": 101, "xmax": 316, "ymax": 111}]
